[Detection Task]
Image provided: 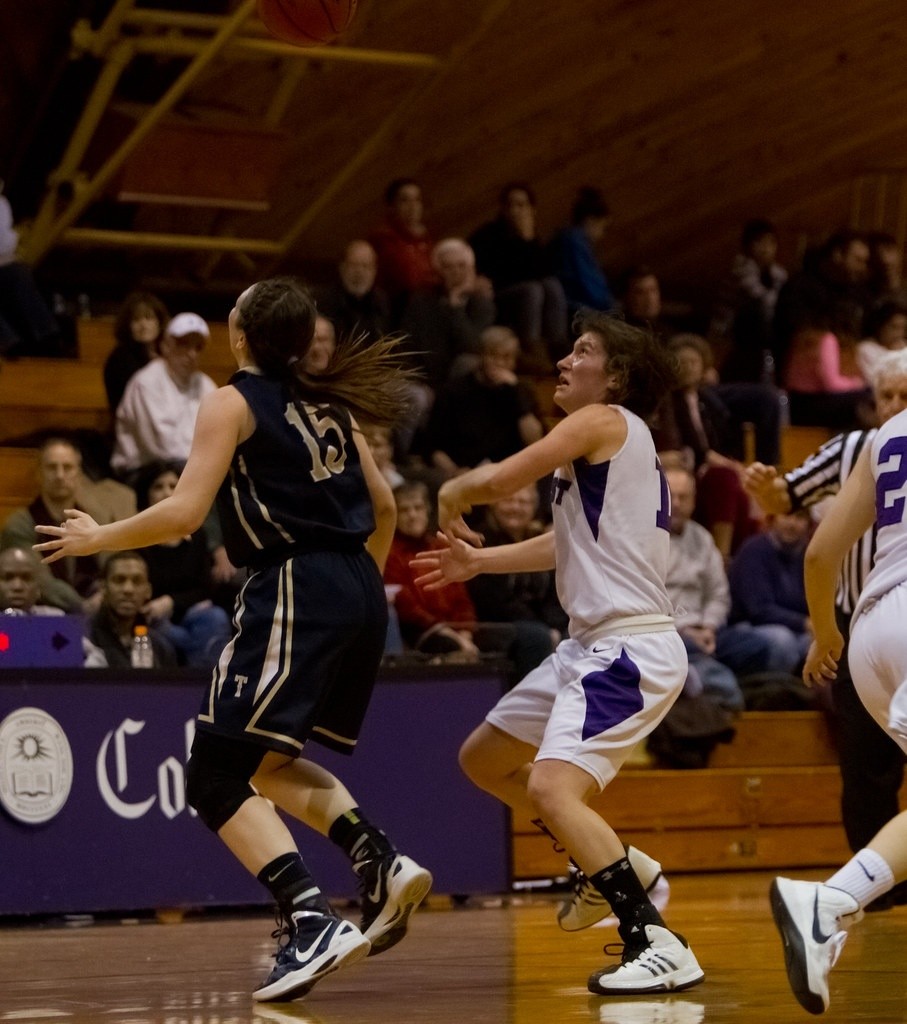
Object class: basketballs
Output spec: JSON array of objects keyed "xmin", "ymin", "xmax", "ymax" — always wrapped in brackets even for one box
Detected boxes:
[{"xmin": 259, "ymin": 1, "xmax": 361, "ymax": 49}]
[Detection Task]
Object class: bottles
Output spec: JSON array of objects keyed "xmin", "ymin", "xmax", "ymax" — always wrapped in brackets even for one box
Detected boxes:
[{"xmin": 131, "ymin": 626, "xmax": 153, "ymax": 668}]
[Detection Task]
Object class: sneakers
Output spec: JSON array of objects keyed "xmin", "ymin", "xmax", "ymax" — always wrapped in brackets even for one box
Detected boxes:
[
  {"xmin": 249, "ymin": 904, "xmax": 371, "ymax": 1004},
  {"xmin": 769, "ymin": 875, "xmax": 863, "ymax": 1015},
  {"xmin": 555, "ymin": 844, "xmax": 665, "ymax": 933},
  {"xmin": 352, "ymin": 849, "xmax": 432, "ymax": 957},
  {"xmin": 585, "ymin": 923, "xmax": 706, "ymax": 994}
]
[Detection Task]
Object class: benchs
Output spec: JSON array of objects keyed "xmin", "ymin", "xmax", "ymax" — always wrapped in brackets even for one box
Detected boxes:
[{"xmin": 0, "ymin": 311, "xmax": 906, "ymax": 880}]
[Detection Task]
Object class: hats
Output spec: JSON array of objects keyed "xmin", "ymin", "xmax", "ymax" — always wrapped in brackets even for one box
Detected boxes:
[{"xmin": 166, "ymin": 310, "xmax": 212, "ymax": 341}]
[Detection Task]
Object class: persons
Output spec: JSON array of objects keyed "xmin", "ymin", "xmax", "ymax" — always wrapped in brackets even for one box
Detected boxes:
[
  {"xmin": 297, "ymin": 177, "xmax": 552, "ymax": 488},
  {"xmin": 465, "ymin": 184, "xmax": 714, "ymax": 375},
  {"xmin": 0, "ymin": 548, "xmax": 107, "ymax": 667},
  {"xmin": 664, "ymin": 465, "xmax": 827, "ymax": 735},
  {"xmin": 103, "ymin": 291, "xmax": 170, "ymax": 434},
  {"xmin": 769, "ymin": 407, "xmax": 907, "ymax": 1014},
  {"xmin": 745, "ymin": 350, "xmax": 907, "ymax": 913},
  {"xmin": 0, "ymin": 178, "xmax": 31, "ymax": 355},
  {"xmin": 409, "ymin": 315, "xmax": 705, "ymax": 995},
  {"xmin": 725, "ymin": 217, "xmax": 907, "ymax": 436},
  {"xmin": 33, "ymin": 281, "xmax": 433, "ymax": 1002},
  {"xmin": 136, "ymin": 459, "xmax": 253, "ymax": 667},
  {"xmin": 90, "ymin": 551, "xmax": 164, "ymax": 668},
  {"xmin": 481, "ymin": 483, "xmax": 569, "ymax": 695},
  {"xmin": 111, "ymin": 312, "xmax": 218, "ymax": 489},
  {"xmin": 382, "ymin": 483, "xmax": 481, "ymax": 665},
  {"xmin": 619, "ymin": 269, "xmax": 745, "ymax": 466},
  {"xmin": 0, "ymin": 440, "xmax": 109, "ymax": 648}
]
